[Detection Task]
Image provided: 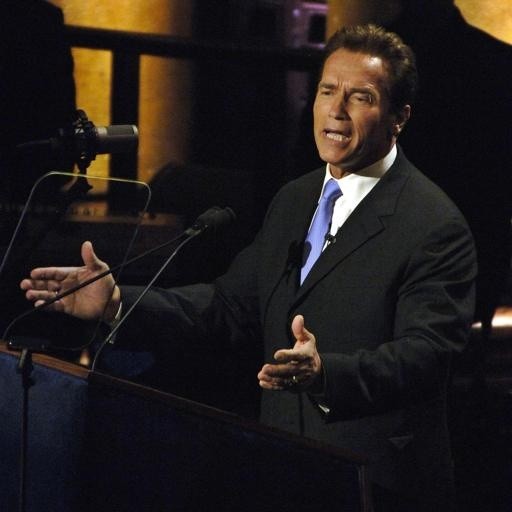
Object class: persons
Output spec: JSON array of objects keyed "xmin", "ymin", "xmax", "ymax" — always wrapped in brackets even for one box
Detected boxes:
[{"xmin": 20, "ymin": 27, "xmax": 480, "ymax": 511}]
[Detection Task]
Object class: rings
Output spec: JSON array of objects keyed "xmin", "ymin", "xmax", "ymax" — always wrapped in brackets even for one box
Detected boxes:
[
  {"xmin": 291, "ymin": 375, "xmax": 298, "ymax": 385},
  {"xmin": 54, "ymin": 290, "xmax": 60, "ymax": 299}
]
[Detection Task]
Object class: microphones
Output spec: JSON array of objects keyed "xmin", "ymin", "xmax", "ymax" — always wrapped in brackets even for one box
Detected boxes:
[
  {"xmin": 37, "ymin": 110, "xmax": 141, "ymax": 174},
  {"xmin": 94, "ymin": 206, "xmax": 237, "ymax": 371},
  {"xmin": 3, "ymin": 200, "xmax": 220, "ymax": 349}
]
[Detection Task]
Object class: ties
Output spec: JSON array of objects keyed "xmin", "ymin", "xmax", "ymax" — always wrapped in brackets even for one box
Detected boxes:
[{"xmin": 297, "ymin": 178, "xmax": 343, "ymax": 285}]
